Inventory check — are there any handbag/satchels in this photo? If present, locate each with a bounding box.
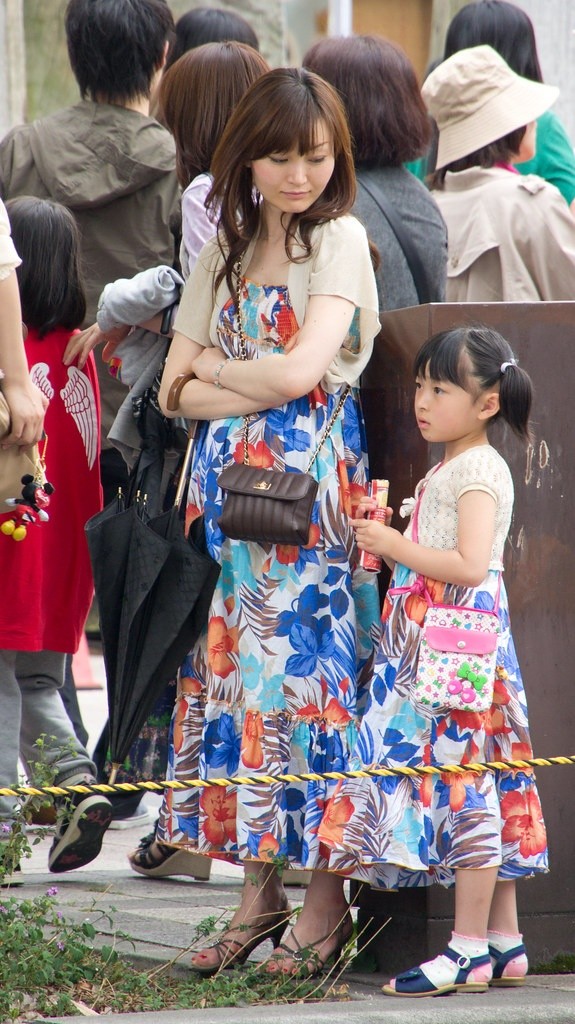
[215,463,319,546]
[414,604,503,712]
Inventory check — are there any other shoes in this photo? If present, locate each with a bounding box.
[0,855,24,885]
[108,801,149,830]
[48,777,113,873]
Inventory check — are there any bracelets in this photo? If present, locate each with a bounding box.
[215,358,233,389]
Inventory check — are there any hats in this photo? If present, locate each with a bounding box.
[419,45,560,174]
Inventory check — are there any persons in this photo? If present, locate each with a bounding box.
[0,0,449,885]
[419,44,575,302]
[401,0,575,208]
[157,67,382,977]
[317,326,550,997]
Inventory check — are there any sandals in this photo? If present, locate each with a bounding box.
[381,943,527,997]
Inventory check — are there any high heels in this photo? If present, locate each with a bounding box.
[129,837,213,881]
[190,895,293,974]
[258,906,354,980]
